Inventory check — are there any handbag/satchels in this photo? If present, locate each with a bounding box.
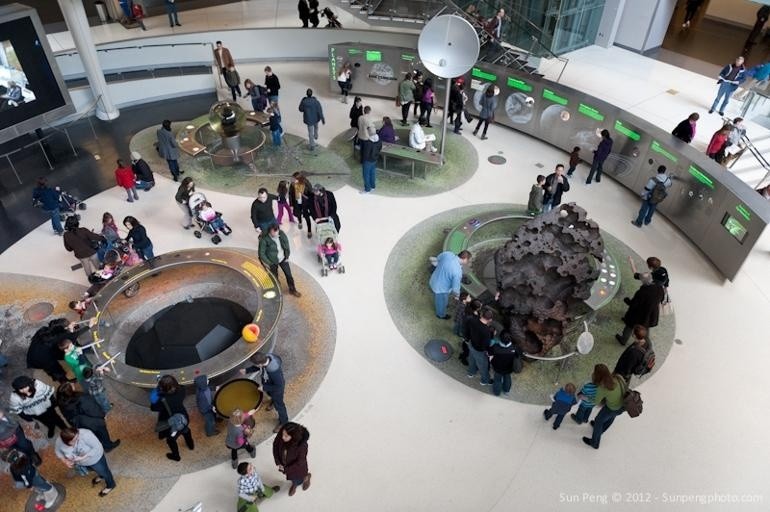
[32,198,44,208]
[659,300,673,317]
[625,388,643,417]
[155,422,169,432]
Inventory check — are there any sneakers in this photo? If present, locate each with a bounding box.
[49,427,55,438]
[459,353,509,396]
[544,408,595,429]
[99,488,112,497]
[437,313,450,318]
[92,476,103,485]
[272,486,280,491]
[207,430,219,436]
[616,334,624,344]
[265,403,273,410]
[273,424,281,433]
[289,219,313,239]
[105,439,120,454]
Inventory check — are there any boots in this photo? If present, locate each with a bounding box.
[36,485,58,509]
[473,128,478,136]
[251,447,255,458]
[232,459,238,469]
[481,133,488,139]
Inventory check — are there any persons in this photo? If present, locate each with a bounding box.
[722,118,744,167]
[744,5,770,53]
[707,125,731,159]
[683,0,703,29]
[709,56,746,116]
[672,113,700,144]
[733,62,770,100]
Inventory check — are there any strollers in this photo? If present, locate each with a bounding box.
[319,7,343,28]
[86,239,141,300]
[184,191,232,244]
[312,215,347,278]
[52,188,88,223]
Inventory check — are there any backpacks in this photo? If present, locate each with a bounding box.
[648,178,667,206]
[637,338,657,376]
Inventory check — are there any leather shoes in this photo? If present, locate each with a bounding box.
[583,437,591,445]
[302,474,311,490]
[166,453,181,461]
[287,484,297,496]
[289,289,300,296]
